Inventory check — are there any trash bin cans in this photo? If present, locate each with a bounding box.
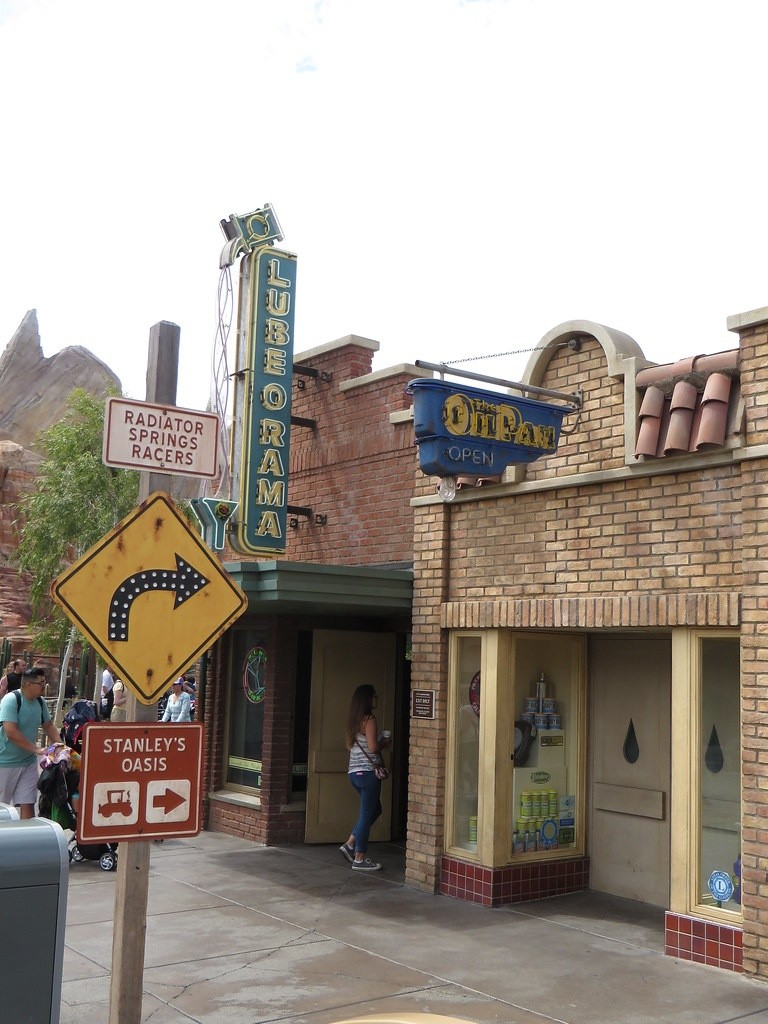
[0,800,68,1024]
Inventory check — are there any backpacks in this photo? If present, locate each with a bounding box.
[100,681,124,718]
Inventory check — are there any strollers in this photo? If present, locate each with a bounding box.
[36,760,118,872]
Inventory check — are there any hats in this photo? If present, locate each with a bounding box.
[174,677,183,684]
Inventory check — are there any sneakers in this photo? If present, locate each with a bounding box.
[339,843,355,864]
[352,858,382,872]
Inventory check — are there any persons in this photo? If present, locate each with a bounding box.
[71,777,80,814]
[339,684,391,870]
[456,687,479,794]
[0,658,199,722]
[0,668,65,819]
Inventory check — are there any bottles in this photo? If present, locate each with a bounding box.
[733,854,741,904]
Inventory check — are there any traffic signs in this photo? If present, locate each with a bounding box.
[76,722,206,845]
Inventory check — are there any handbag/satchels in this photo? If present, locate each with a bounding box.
[375,765,389,778]
[37,768,57,795]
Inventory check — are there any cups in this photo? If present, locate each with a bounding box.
[382,731,391,738]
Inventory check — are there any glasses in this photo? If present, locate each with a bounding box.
[374,695,378,698]
[29,680,46,686]
